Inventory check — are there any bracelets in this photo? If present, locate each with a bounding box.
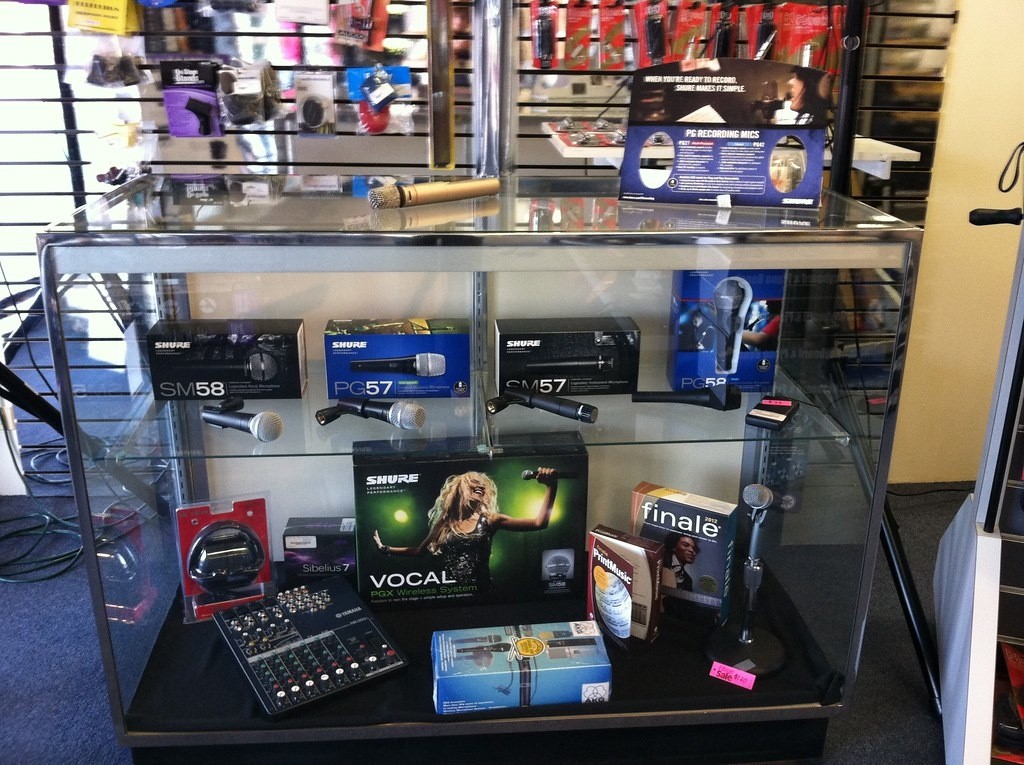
[381,545,390,555]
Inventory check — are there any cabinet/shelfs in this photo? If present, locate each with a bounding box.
[38,0,924,765]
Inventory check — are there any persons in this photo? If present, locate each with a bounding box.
[787,64,828,124]
[372,466,559,593]
[661,530,700,592]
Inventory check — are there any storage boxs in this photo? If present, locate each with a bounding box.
[153,319,310,401]
[431,618,610,715]
[323,316,470,401]
[665,268,788,393]
[587,524,667,643]
[283,518,356,577]
[618,57,829,213]
[495,315,642,399]
[630,479,740,628]
[352,428,589,617]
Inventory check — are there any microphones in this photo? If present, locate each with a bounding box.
[522,470,582,480]
[743,484,782,509]
[457,643,511,653]
[336,396,424,430]
[712,276,753,374]
[351,352,446,377]
[201,406,283,442]
[527,355,613,374]
[502,386,598,423]
[174,353,278,380]
[452,635,501,646]
[367,177,500,209]
[761,80,778,120]
[699,576,716,597]
[632,384,742,411]
